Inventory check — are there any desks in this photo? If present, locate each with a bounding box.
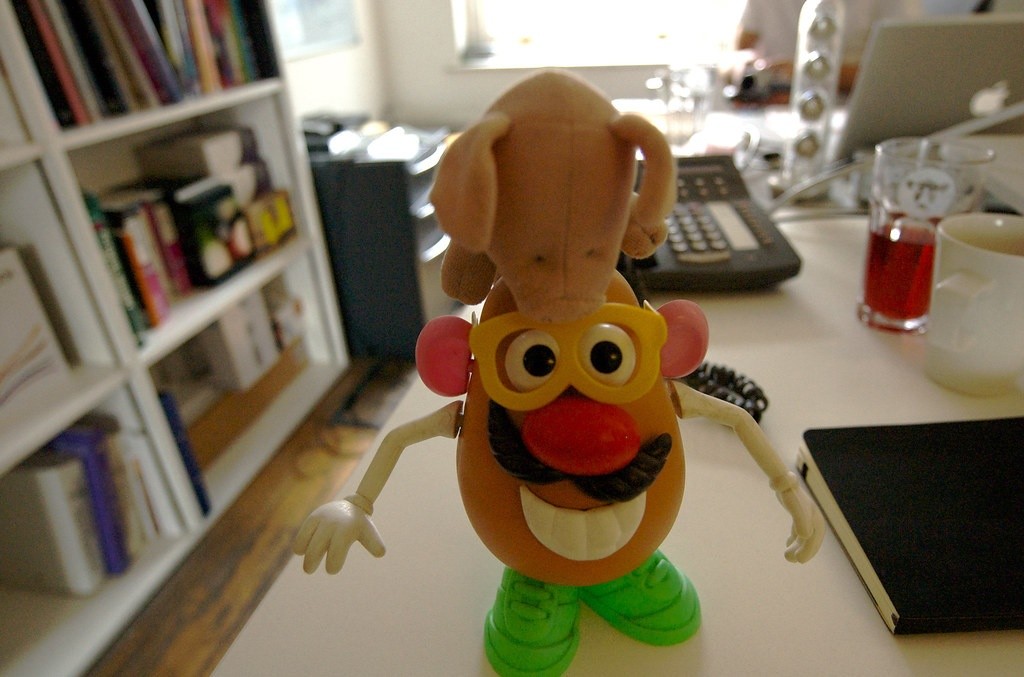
[206,98,1023,676]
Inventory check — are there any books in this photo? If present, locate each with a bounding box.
[1,0,297,601]
[796,416,1024,635]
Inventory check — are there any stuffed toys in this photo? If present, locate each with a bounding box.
[424,69,679,320]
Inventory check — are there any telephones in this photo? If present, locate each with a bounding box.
[613,155,800,306]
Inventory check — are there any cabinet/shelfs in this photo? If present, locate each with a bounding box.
[1,1,350,676]
[309,127,451,362]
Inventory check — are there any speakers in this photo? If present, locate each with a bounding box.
[777,0,847,195]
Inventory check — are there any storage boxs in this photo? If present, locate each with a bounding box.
[185,340,311,469]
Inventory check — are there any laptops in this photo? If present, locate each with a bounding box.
[770,13,1024,164]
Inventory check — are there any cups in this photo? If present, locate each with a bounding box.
[855,134,996,336]
[923,213,1024,397]
